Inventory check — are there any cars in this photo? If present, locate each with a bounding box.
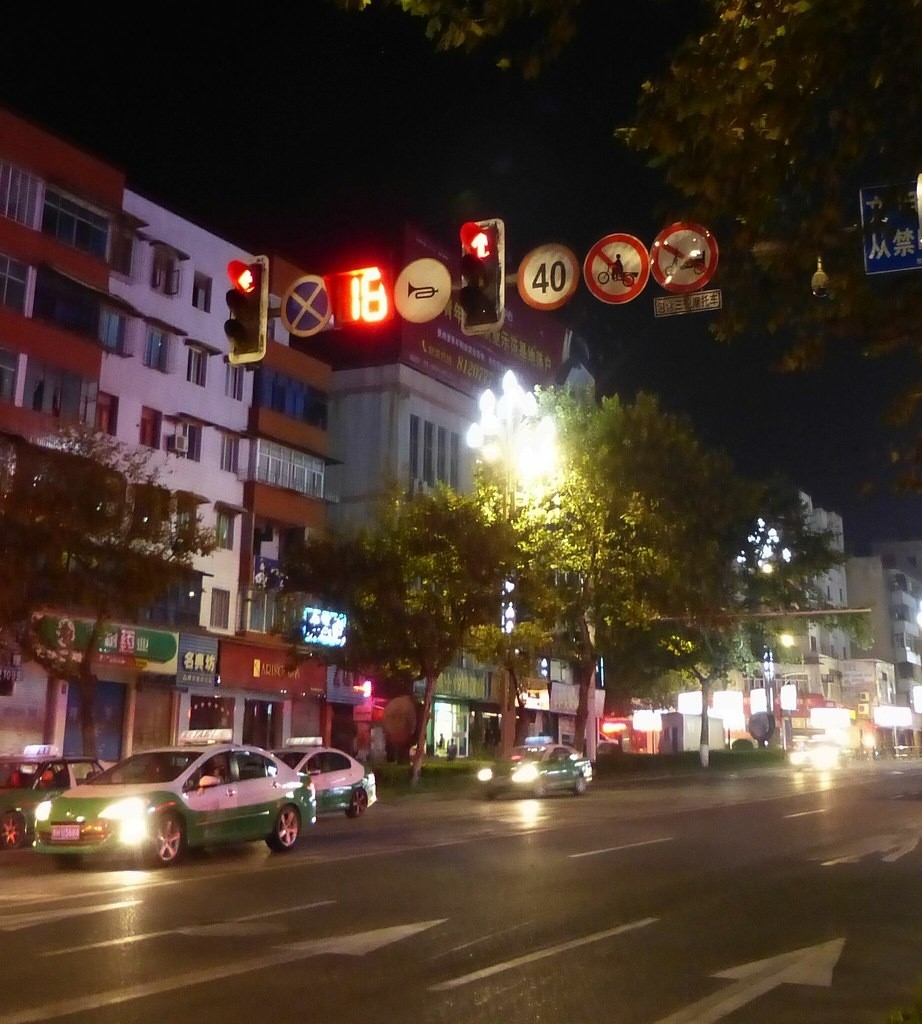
[793,741,847,770]
[478,735,592,798]
[264,736,377,818]
[32,728,318,868]
[0,745,108,850]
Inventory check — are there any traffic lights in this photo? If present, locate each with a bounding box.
[333,258,391,325]
[459,218,505,336]
[224,255,269,364]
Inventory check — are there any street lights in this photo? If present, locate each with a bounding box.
[737,519,794,747]
[467,369,561,749]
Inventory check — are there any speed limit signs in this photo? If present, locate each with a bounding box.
[518,243,580,311]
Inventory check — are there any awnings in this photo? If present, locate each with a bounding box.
[149,237,190,263]
[214,501,248,516]
[144,314,188,337]
[183,338,224,357]
[50,170,149,232]
[175,489,211,503]
[176,412,254,440]
[38,261,144,318]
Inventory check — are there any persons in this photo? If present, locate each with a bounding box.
[445,738,457,760]
[36,766,59,789]
